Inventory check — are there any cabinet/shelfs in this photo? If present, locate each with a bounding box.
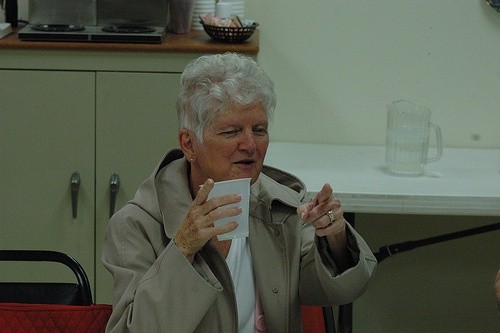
[0,53,258,309]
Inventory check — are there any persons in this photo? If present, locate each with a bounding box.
[102,51,378,333]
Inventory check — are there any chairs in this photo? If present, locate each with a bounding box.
[0,248,113,333]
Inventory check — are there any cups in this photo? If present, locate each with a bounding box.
[198,178,251,241]
[192,0,245,30]
[168,0,194,34]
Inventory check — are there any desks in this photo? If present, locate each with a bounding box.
[264,141,500,333]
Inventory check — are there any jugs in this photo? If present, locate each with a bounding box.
[384,97,443,177]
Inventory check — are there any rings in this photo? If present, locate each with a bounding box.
[326,209,337,224]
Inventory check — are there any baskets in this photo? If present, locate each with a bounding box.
[199,20,256,42]
